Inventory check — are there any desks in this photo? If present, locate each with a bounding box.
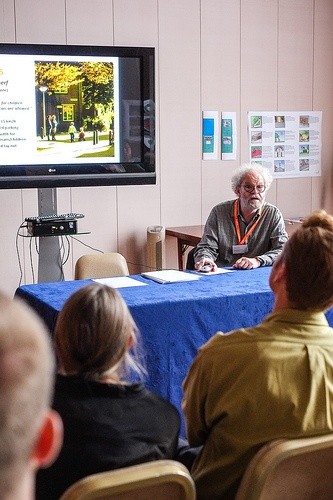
[166,223,302,271]
[14,265,333,441]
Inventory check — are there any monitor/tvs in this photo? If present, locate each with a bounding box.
[0,43,157,190]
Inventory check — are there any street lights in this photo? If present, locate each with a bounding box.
[39,86,48,138]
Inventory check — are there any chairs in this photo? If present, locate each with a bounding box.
[60,459,196,500]
[235,434,333,500]
[75,253,129,280]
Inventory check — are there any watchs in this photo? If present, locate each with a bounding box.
[257,257,263,267]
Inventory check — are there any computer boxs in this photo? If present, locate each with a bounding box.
[26,220,78,236]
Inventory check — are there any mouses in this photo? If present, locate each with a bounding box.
[201,265,212,272]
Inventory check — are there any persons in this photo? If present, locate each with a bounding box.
[46,114,87,143]
[177,210,333,500]
[194,162,288,270]
[0,284,181,500]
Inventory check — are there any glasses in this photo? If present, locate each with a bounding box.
[240,185,267,193]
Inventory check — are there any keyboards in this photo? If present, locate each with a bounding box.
[25,214,85,222]
